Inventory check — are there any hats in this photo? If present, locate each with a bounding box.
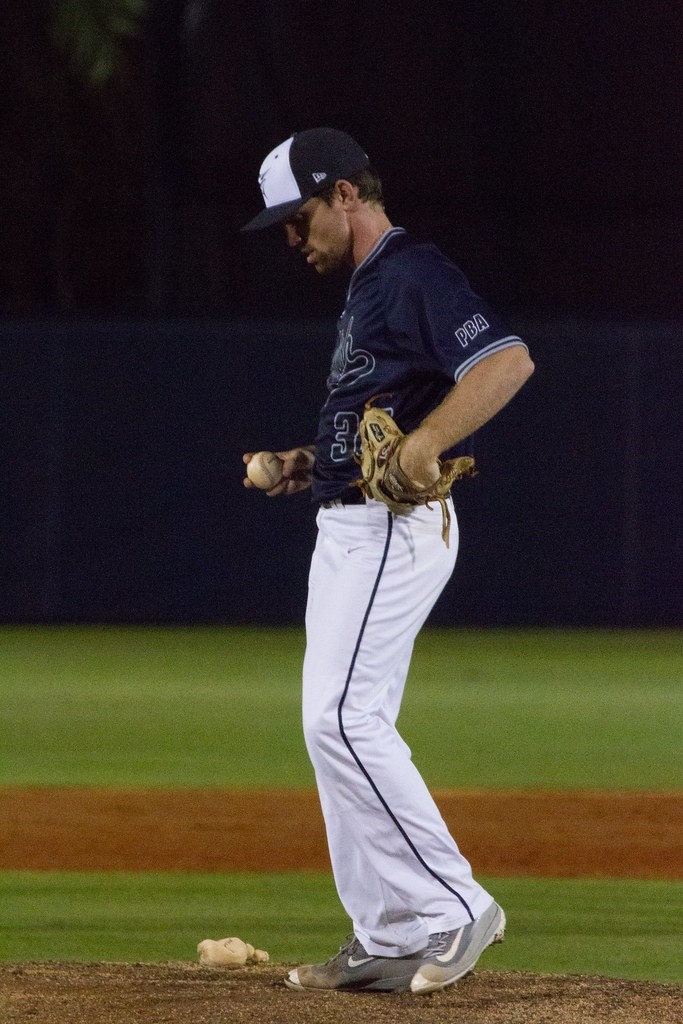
[240,128,370,232]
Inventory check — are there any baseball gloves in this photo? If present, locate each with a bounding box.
[356,406,477,518]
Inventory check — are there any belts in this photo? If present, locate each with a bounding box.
[322,494,418,508]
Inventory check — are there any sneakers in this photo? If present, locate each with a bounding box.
[283,933,427,994]
[411,901,507,994]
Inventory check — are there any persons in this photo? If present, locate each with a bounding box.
[243,128,538,998]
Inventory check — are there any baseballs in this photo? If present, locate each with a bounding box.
[246,450,285,491]
